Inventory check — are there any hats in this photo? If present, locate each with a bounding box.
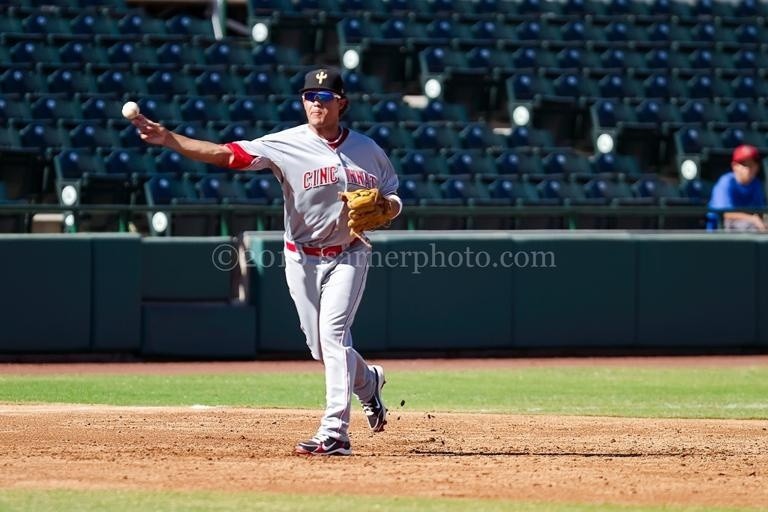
[733,145,759,162]
[299,69,344,97]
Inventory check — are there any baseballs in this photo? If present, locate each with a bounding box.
[120,101,139,120]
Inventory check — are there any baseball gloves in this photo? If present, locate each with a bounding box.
[337,188,393,234]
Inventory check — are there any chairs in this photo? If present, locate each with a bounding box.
[0,0,768,205]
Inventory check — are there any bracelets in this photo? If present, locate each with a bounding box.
[387,195,403,220]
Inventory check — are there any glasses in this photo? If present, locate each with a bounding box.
[304,91,340,101]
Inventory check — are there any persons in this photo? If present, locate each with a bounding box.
[705,144,768,231]
[131,67,403,457]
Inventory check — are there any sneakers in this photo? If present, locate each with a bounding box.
[295,431,352,455]
[360,364,386,431]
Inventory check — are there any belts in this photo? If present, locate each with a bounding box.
[286,236,359,257]
